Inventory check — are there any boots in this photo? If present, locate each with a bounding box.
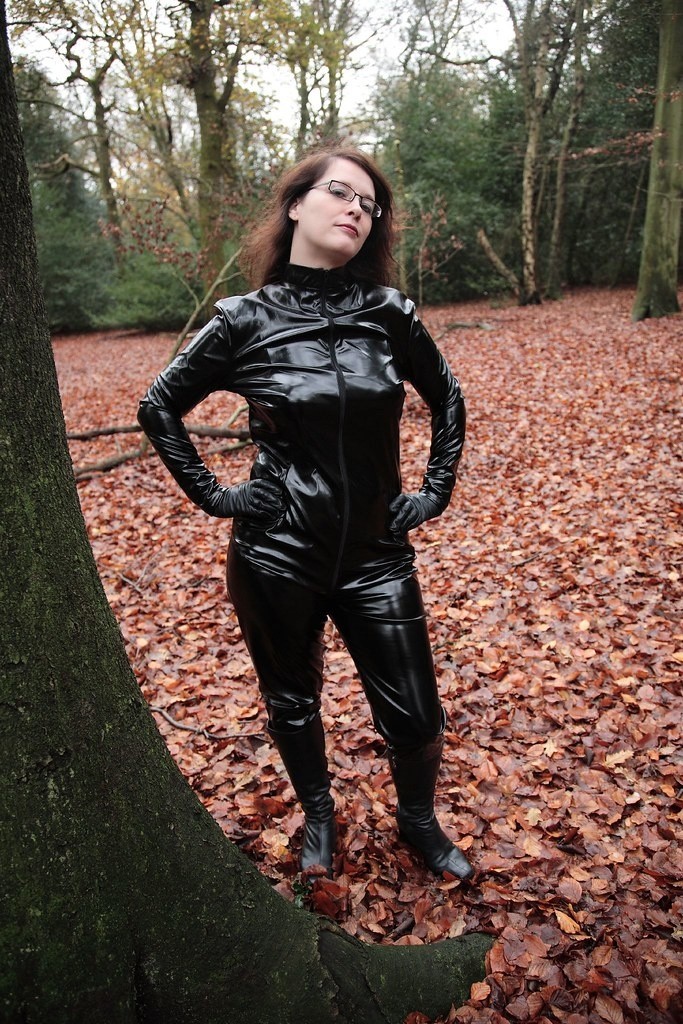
[265,712,335,889]
[385,742,474,880]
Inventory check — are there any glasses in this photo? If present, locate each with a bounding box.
[303,180,382,217]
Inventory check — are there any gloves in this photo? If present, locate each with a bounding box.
[388,489,445,541]
[211,479,288,522]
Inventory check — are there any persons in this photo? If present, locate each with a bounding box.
[136,149,476,883]
